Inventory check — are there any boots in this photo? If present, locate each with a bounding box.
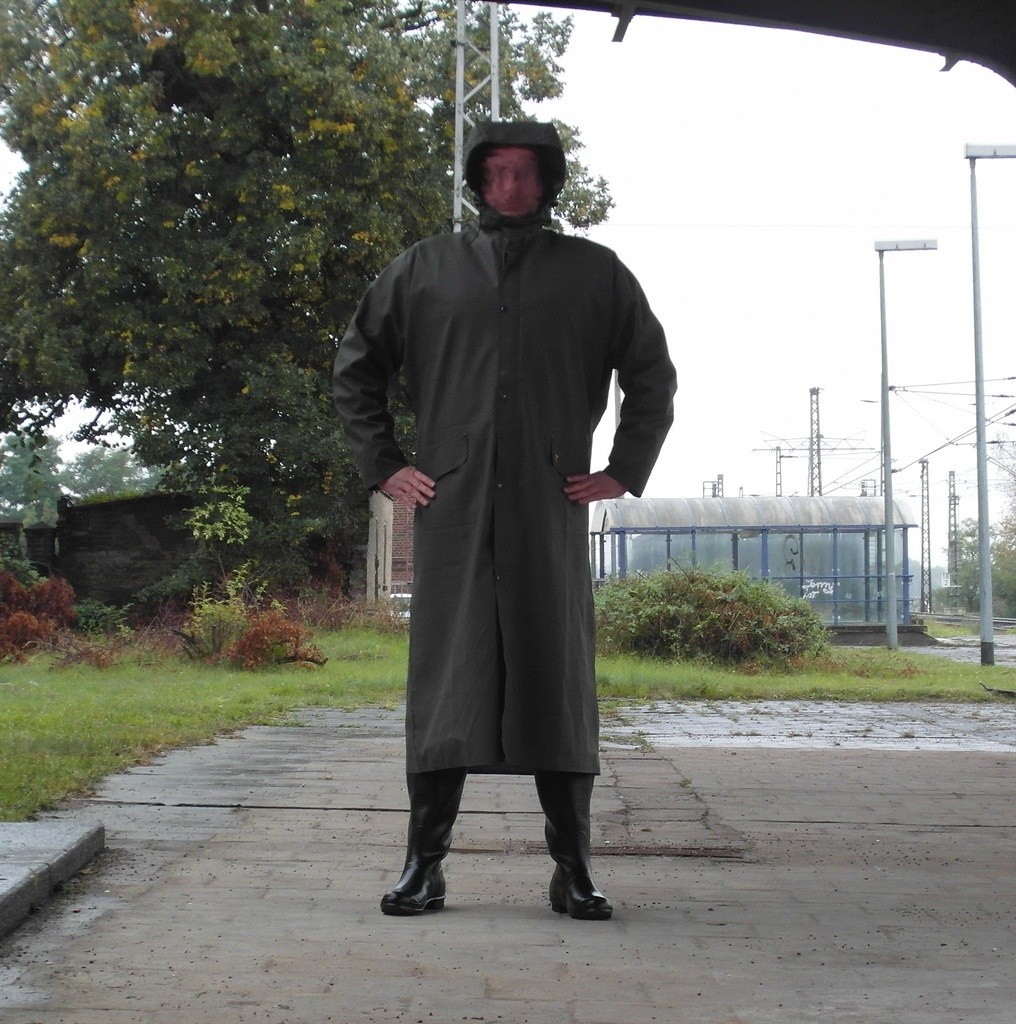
[535,772,613,919]
[381,768,469,916]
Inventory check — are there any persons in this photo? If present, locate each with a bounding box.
[333,121,678,920]
